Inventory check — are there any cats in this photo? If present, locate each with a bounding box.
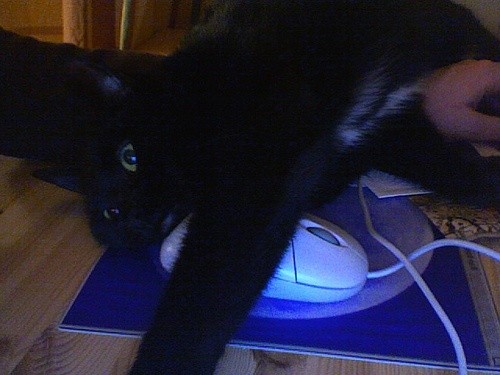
[31,0,499,375]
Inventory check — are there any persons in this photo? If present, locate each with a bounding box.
[1,25,500,170]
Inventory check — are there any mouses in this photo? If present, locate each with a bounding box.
[160,211,369,304]
